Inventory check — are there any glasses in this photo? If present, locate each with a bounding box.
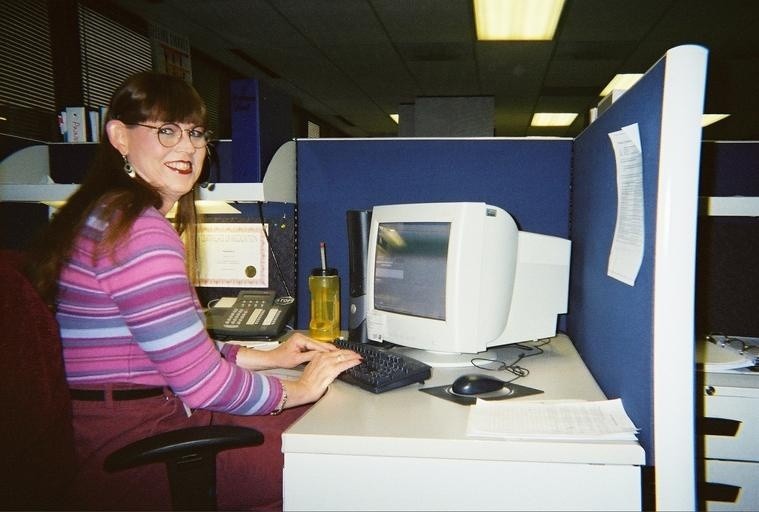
[124,121,209,149]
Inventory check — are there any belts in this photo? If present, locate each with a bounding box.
[69,385,173,402]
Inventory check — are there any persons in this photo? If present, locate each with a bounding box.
[24,68,362,512]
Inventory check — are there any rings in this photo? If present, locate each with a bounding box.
[336,354,343,361]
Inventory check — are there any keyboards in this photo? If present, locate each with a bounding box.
[301,339,431,394]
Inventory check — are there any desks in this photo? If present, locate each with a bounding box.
[253,326,645,511]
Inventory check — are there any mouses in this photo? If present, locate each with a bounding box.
[453,377,505,396]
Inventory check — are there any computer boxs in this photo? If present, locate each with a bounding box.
[346,209,374,344]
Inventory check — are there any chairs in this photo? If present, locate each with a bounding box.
[1,250,264,512]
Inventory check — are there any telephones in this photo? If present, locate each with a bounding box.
[205,290,297,339]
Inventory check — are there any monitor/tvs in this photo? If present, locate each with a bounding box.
[367,202,571,368]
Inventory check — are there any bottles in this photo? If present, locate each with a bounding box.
[308,268,340,341]
